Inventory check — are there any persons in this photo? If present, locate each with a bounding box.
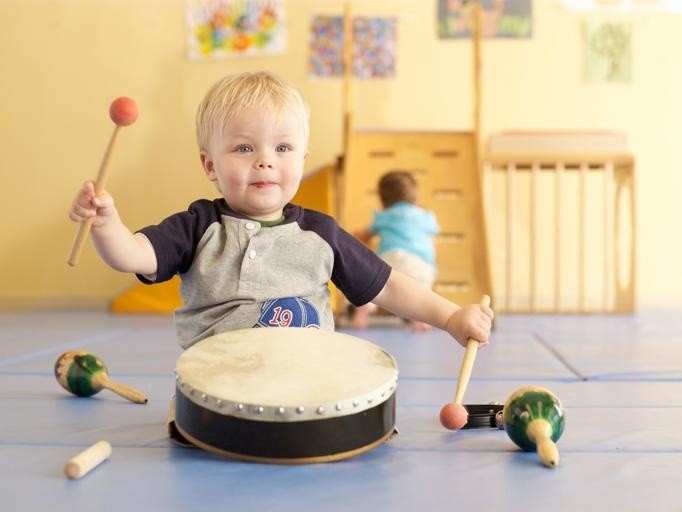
[69,71,494,448]
[345,171,442,332]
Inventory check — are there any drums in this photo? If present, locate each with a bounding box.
[176,327,397,462]
